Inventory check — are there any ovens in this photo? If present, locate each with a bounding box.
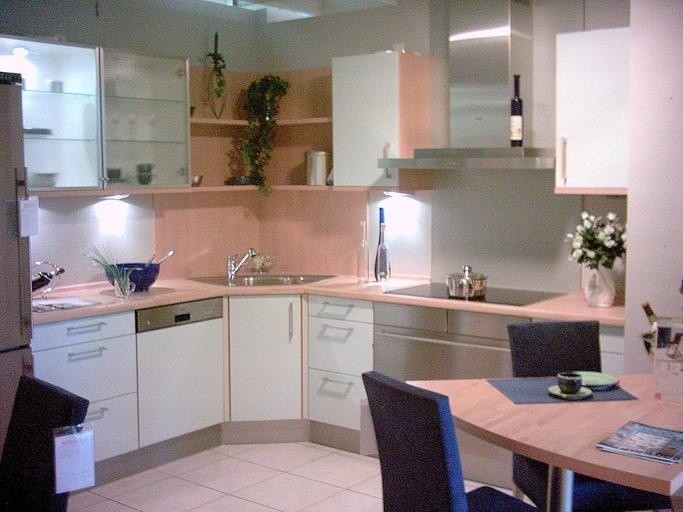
[374,303,532,489]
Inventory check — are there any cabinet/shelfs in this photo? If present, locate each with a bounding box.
[31,310,138,464]
[305,297,376,431]
[227,294,303,422]
[191,69,332,195]
[554,28,628,195]
[100,46,191,190]
[332,52,451,191]
[0,33,102,192]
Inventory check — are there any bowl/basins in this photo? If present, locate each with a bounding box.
[192,175,203,187]
[28,170,60,186]
[104,262,162,293]
[245,254,279,276]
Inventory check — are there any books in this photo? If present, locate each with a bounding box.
[593,418,683,467]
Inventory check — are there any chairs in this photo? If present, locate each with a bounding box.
[0,374,90,511]
[361,370,541,512]
[506,320,612,512]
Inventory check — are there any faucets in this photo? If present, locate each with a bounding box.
[223,246,257,286]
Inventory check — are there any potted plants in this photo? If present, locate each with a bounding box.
[228,74,291,199]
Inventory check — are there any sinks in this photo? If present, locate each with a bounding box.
[236,273,293,286]
[294,272,336,286]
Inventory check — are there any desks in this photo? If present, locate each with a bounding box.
[404,376,683,512]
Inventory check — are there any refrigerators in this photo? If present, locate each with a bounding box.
[1,71,36,456]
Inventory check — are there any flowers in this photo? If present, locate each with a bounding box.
[563,210,628,269]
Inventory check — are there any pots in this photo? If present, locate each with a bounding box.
[444,263,489,302]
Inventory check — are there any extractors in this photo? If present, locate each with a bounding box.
[376,1,557,173]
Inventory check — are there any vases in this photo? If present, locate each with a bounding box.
[584,265,616,308]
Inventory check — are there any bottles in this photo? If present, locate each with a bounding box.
[641,301,663,333]
[509,73,524,147]
[31,264,66,293]
[355,219,371,286]
[375,207,392,285]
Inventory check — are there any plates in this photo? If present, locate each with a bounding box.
[573,370,620,392]
[547,384,593,401]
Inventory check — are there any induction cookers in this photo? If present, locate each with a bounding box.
[384,281,570,307]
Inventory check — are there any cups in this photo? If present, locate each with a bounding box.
[305,150,330,186]
[106,159,159,185]
[557,370,582,395]
[50,80,63,93]
